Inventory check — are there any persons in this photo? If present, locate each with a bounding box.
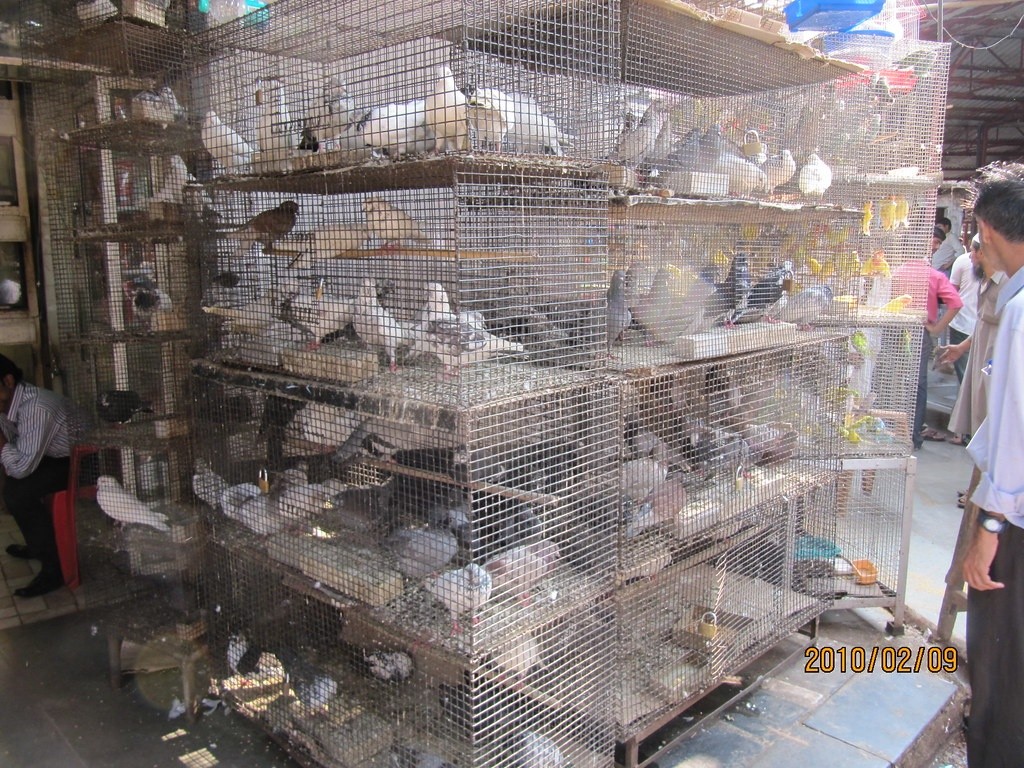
[963,162,1024,766]
[0,354,73,599]
[838,215,1011,515]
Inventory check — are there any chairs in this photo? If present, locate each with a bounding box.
[53,444,99,589]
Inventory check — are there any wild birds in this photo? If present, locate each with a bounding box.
[668,190,918,447]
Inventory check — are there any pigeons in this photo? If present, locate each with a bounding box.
[91,58,842,729]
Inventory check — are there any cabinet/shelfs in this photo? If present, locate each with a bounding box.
[0,55,110,417]
[182,0,618,768]
[17,0,235,663]
[613,0,847,741]
[694,28,953,635]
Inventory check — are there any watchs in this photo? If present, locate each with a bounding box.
[976,508,1006,535]
